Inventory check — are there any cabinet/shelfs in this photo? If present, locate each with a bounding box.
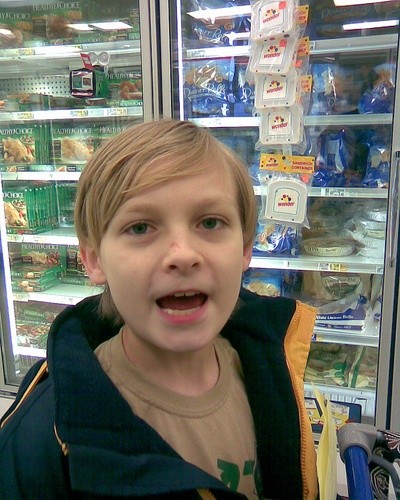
[0,0,400,444]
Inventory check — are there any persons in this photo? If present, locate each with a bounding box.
[0,119,319,500]
